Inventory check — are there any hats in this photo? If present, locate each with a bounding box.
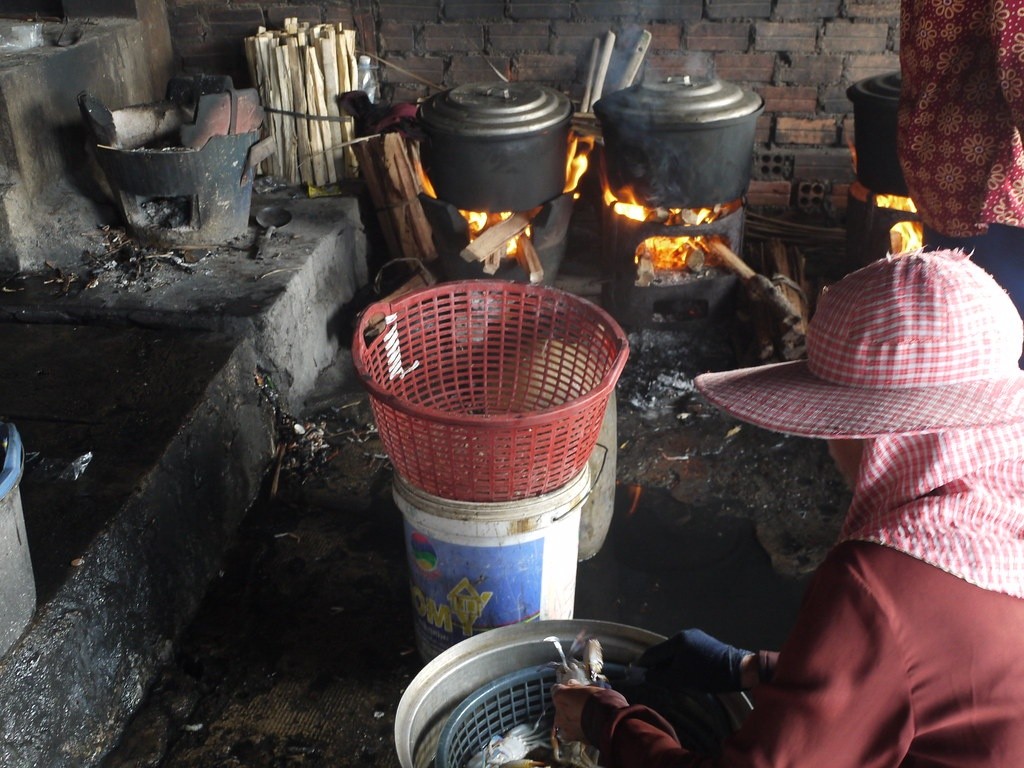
[693,246,1024,440]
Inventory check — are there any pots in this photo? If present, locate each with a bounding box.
[418,77,572,213]
[589,71,765,212]
[843,71,931,198]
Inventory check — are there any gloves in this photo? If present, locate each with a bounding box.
[636,627,753,699]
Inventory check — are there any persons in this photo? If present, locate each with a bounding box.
[552,245,1024,768]
[900,0,1024,370]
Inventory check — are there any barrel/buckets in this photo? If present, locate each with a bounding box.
[389,438,614,677]
[392,620,773,768]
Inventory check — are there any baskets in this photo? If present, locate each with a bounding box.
[352,277,632,502]
[434,661,630,768]
